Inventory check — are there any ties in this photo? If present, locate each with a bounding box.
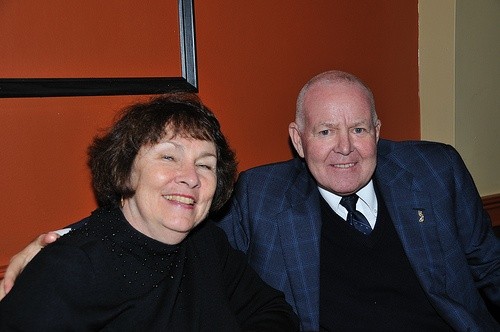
[338,193,374,236]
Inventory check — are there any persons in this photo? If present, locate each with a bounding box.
[0,71,500,331]
[0,89,305,332]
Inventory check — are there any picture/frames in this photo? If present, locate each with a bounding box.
[0,0,201,98]
